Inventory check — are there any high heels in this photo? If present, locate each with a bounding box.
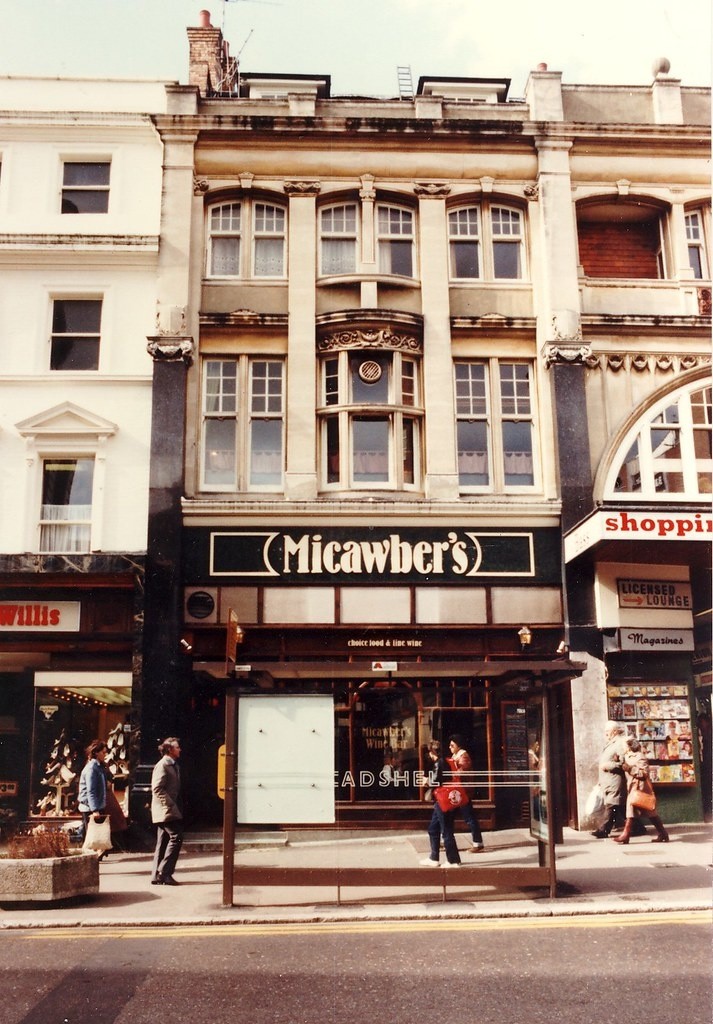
[652,833,669,842]
[614,834,629,844]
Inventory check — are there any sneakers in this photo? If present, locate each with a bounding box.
[441,861,459,868]
[419,858,438,866]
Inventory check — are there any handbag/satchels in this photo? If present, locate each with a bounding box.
[82,814,112,850]
[433,780,469,812]
[587,784,605,813]
[631,778,656,811]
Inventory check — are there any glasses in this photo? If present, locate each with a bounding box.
[449,744,459,747]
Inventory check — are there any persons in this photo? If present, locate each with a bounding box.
[426,741,461,872]
[528,740,544,821]
[81,740,114,862]
[631,720,692,759]
[384,740,402,774]
[149,736,185,889]
[615,740,670,847]
[591,722,646,840]
[440,736,485,853]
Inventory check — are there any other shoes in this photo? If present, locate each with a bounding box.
[151,871,180,885]
[612,827,623,832]
[631,825,647,835]
[439,843,446,851]
[591,829,607,838]
[469,846,483,852]
